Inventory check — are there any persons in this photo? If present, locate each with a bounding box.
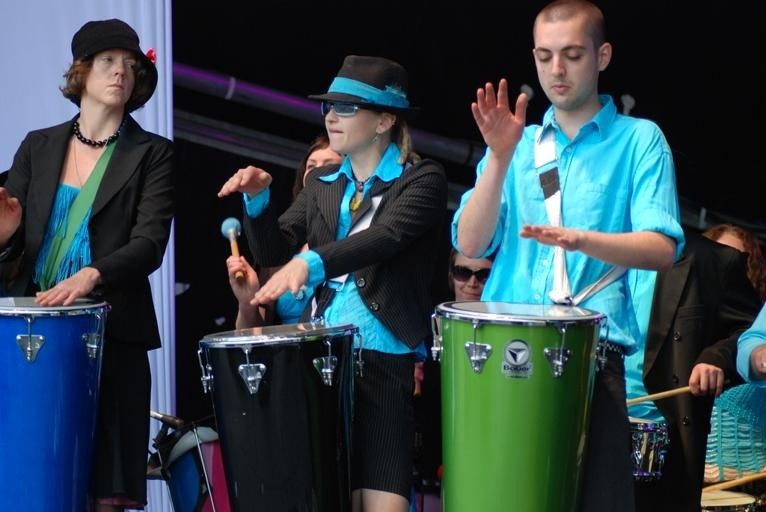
[641,221,766,512]
[414,247,497,486]
[445,2,687,510]
[0,18,188,508]
[215,52,446,512]
[291,132,342,200]
[226,241,314,331]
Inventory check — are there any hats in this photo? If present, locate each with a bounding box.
[309,55,408,110]
[71,18,158,114]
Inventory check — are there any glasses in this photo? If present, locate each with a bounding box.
[319,101,385,117]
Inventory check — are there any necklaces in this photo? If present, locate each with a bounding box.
[72,117,128,147]
[352,172,370,211]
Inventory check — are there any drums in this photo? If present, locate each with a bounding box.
[702,487,758,512]
[201,323,352,512]
[0,296,107,512]
[628,415,668,482]
[436,302,605,511]
